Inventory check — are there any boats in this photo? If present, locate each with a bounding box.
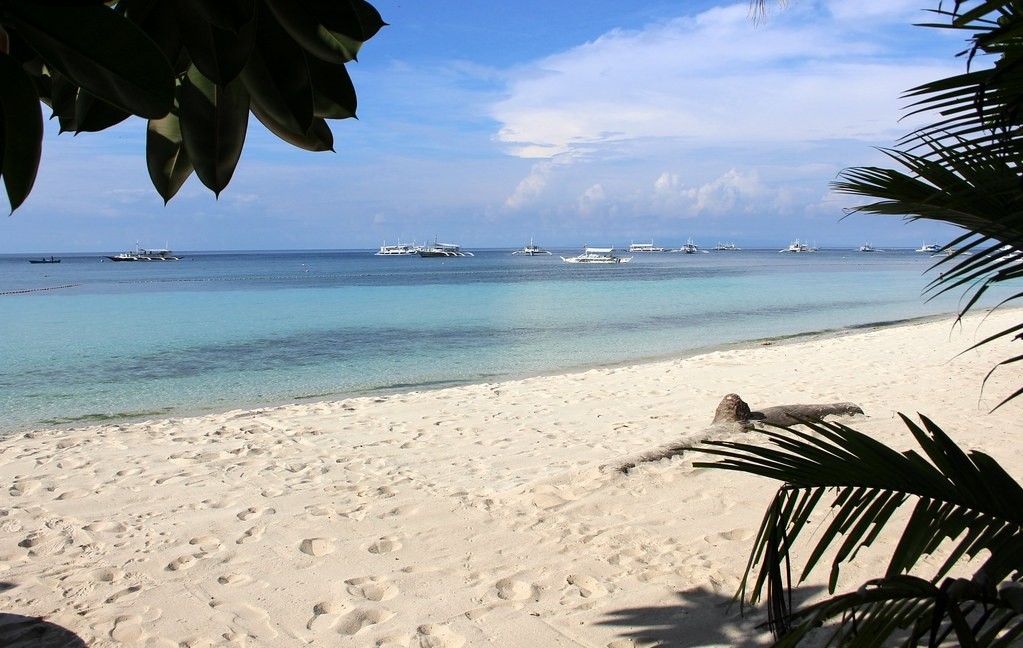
[916,241,942,252]
[374,234,475,257]
[778,236,819,253]
[711,240,742,251]
[103,240,184,261]
[511,237,553,256]
[671,235,699,254]
[29,260,61,263]
[560,243,633,264]
[627,239,665,252]
[854,245,876,252]
